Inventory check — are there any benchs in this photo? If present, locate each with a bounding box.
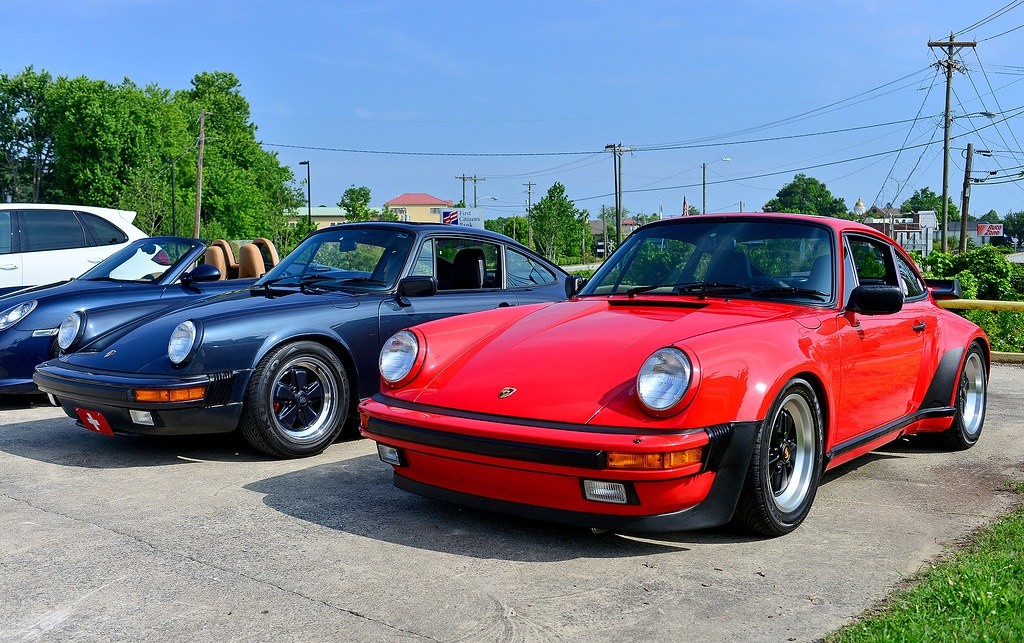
[776,278,887,291]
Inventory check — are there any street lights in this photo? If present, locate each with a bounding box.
[298,159,312,234]
[473,197,498,208]
[604,143,622,251]
[702,157,730,216]
[938,112,996,252]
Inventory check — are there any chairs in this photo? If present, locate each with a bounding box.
[701,252,754,291]
[452,249,487,290]
[238,244,265,278]
[205,246,227,280]
[385,251,408,285]
[804,255,832,301]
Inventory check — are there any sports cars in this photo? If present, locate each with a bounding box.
[32,222,589,462]
[364,210,993,540]
[0,237,352,407]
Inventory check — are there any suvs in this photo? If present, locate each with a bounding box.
[0,201,183,291]
